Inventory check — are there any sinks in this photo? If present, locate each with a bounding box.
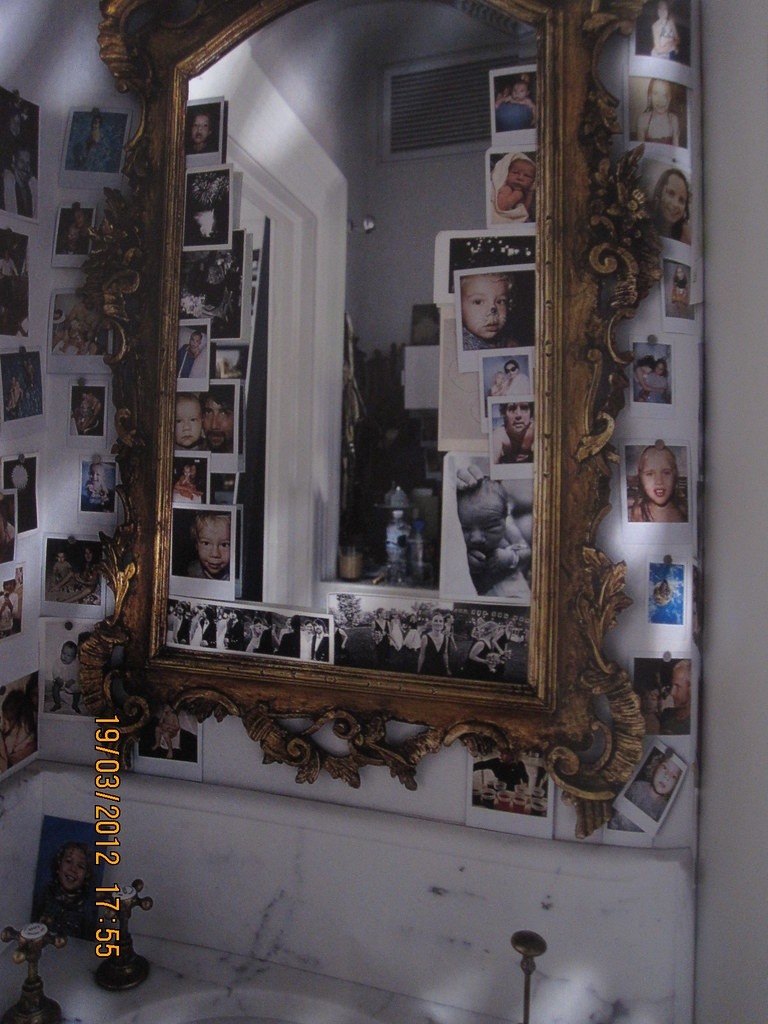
[113,989,381,1024]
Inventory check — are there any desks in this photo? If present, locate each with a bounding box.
[337,553,435,590]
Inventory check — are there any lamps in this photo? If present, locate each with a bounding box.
[347,213,374,236]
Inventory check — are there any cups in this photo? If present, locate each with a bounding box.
[338,547,361,582]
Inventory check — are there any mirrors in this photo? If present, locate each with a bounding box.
[76,0,664,838]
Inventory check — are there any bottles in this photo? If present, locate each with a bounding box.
[382,476,430,584]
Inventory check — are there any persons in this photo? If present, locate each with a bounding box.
[76,389,101,437]
[5,356,36,411]
[638,0,690,320]
[0,250,20,321]
[52,298,106,357]
[184,113,215,156]
[31,831,104,940]
[0,673,41,774]
[61,209,91,254]
[50,641,83,713]
[624,553,691,822]
[0,107,38,221]
[173,328,236,587]
[75,116,112,172]
[168,599,528,685]
[84,464,110,512]
[456,464,531,592]
[0,495,23,638]
[51,546,101,606]
[152,704,180,758]
[634,356,670,403]
[626,445,687,523]
[461,75,538,350]
[474,752,529,792]
[491,360,534,464]
